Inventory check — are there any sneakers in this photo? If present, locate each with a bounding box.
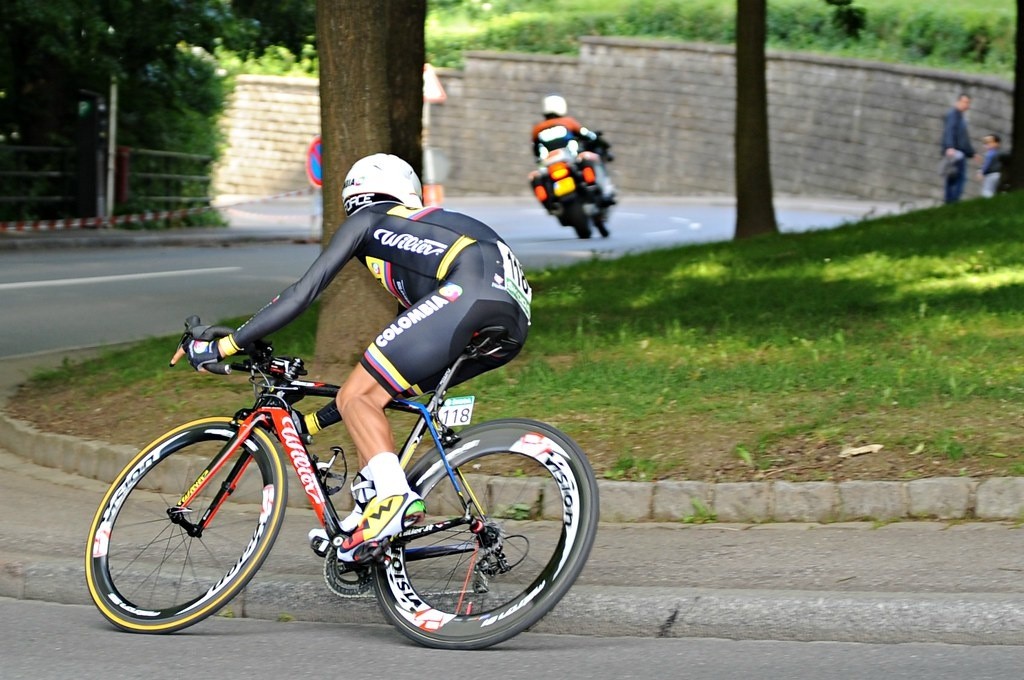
[307,503,368,545]
[335,491,426,566]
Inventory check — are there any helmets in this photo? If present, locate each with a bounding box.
[341,153,423,216]
[541,96,568,118]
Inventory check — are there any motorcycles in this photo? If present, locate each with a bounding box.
[527,125,619,241]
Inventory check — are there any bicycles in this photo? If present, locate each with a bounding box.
[84,315,600,651]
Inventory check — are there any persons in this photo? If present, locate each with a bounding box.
[974,134,1005,197]
[532,95,616,203]
[941,92,975,204]
[169,154,533,563]
